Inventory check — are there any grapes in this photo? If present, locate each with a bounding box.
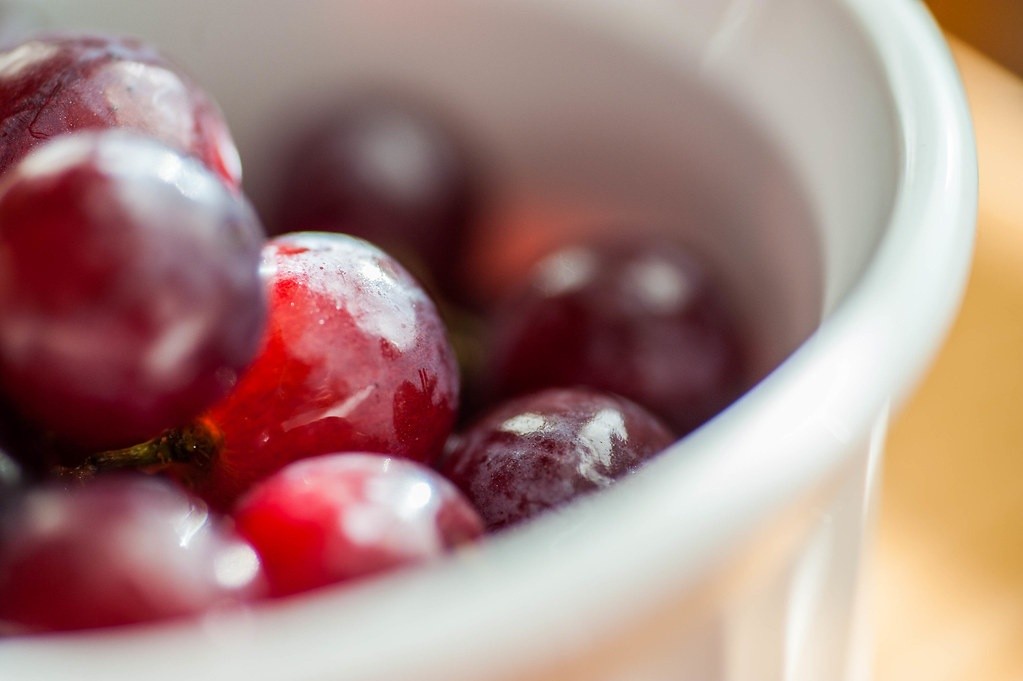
[0,40,736,640]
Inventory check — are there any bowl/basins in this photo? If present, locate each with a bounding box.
[1,1,979,681]
